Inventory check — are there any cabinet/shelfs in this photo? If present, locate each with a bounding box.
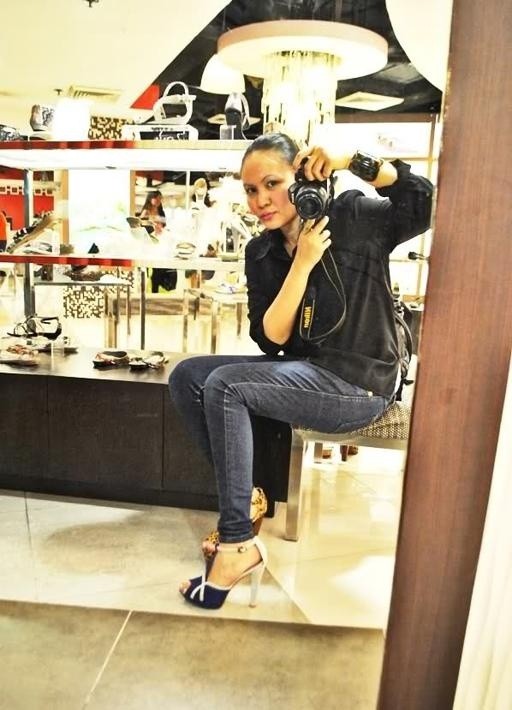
[311,112,440,296]
[0,140,292,518]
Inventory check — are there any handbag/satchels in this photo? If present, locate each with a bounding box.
[135,213,154,235]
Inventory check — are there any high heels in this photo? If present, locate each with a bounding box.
[201,488,268,563]
[179,536,267,610]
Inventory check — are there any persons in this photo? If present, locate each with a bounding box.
[170,132,434,606]
[139,191,166,233]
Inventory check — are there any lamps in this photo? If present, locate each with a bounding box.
[217,19,389,146]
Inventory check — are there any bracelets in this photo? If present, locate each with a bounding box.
[348,152,381,181]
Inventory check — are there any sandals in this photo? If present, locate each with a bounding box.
[6,211,59,254]
[7,313,62,341]
[214,271,248,295]
[32,336,79,354]
[0,344,43,367]
[190,178,208,218]
[223,90,250,139]
[92,350,169,372]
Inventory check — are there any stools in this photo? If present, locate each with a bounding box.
[282,400,415,543]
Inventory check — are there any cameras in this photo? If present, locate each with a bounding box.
[288,157,328,228]
